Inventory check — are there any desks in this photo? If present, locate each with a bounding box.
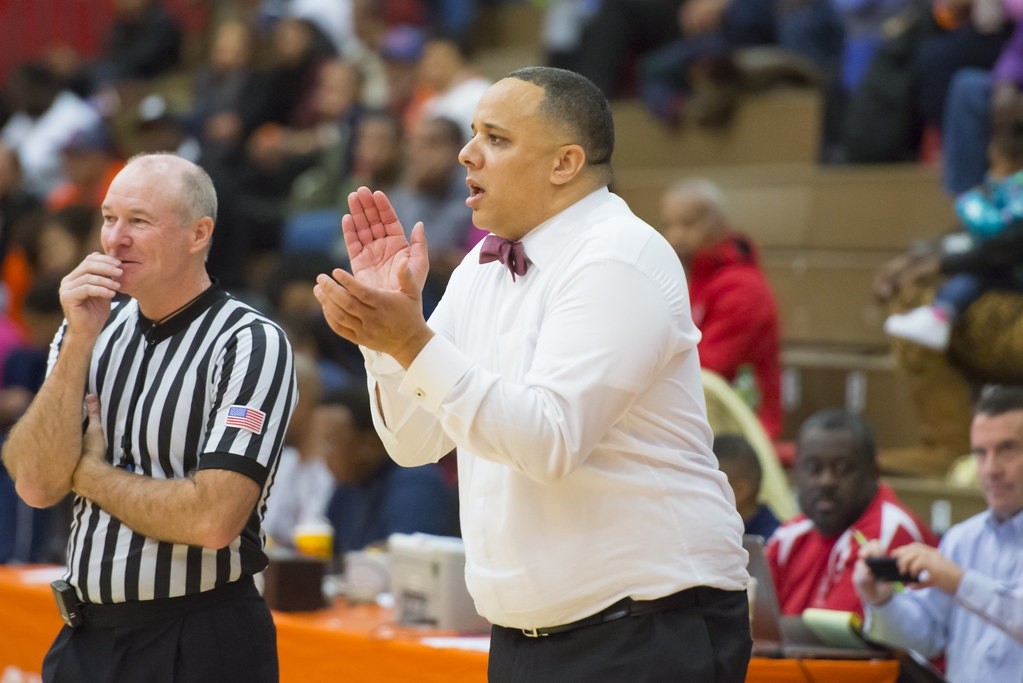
[0,564,898,683]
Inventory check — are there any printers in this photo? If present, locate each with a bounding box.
[387,532,492,635]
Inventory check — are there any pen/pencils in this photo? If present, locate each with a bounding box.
[855,531,904,593]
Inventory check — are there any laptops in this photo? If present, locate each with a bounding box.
[742,534,891,659]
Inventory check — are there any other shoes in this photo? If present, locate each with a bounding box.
[884,302,961,353]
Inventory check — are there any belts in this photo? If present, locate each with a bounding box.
[520,592,733,638]
[78,578,255,633]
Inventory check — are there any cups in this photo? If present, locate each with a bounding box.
[292,527,336,570]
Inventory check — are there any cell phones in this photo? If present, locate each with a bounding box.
[866,555,919,584]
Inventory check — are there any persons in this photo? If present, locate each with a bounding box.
[850,385,1023,683]
[530,0,1023,357]
[711,433,779,542]
[759,405,946,674]
[664,178,783,440]
[312,66,753,683]
[0,152,300,683]
[0,0,495,571]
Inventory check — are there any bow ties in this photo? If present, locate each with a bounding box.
[479,235,529,282]
